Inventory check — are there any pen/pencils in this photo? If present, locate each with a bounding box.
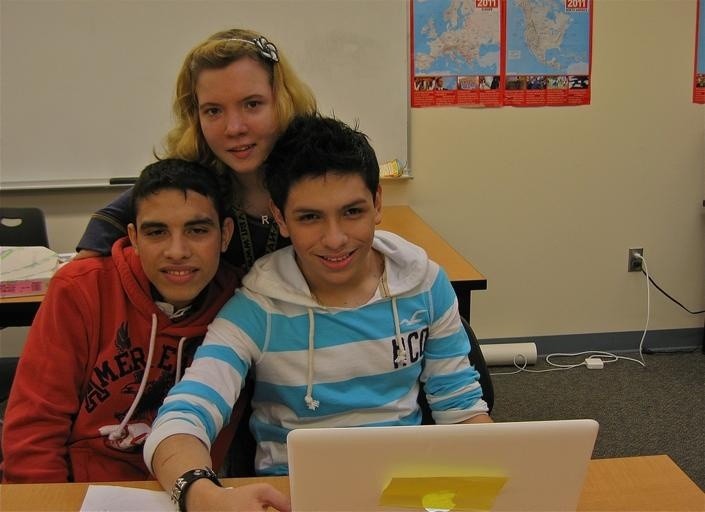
[109,177,138,185]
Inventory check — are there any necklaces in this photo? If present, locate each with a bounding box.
[244,200,271,225]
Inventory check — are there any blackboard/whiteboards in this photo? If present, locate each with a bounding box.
[0,0,412,190]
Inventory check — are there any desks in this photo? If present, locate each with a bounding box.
[0,453,705,512]
[0,205,488,326]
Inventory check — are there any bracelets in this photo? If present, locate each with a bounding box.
[169,465,222,511]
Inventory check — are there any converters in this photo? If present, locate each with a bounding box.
[584,357,604,371]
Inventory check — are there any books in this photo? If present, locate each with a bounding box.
[0,246,60,298]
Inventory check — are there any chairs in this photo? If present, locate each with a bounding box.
[417,314,493,425]
[0,207,49,329]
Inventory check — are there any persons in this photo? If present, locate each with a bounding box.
[414,77,437,91]
[0,152,251,486]
[69,29,320,273]
[140,112,495,511]
[435,77,449,91]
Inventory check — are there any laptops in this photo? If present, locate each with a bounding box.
[285,418,602,512]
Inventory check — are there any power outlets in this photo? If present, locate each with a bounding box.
[628,248,643,273]
